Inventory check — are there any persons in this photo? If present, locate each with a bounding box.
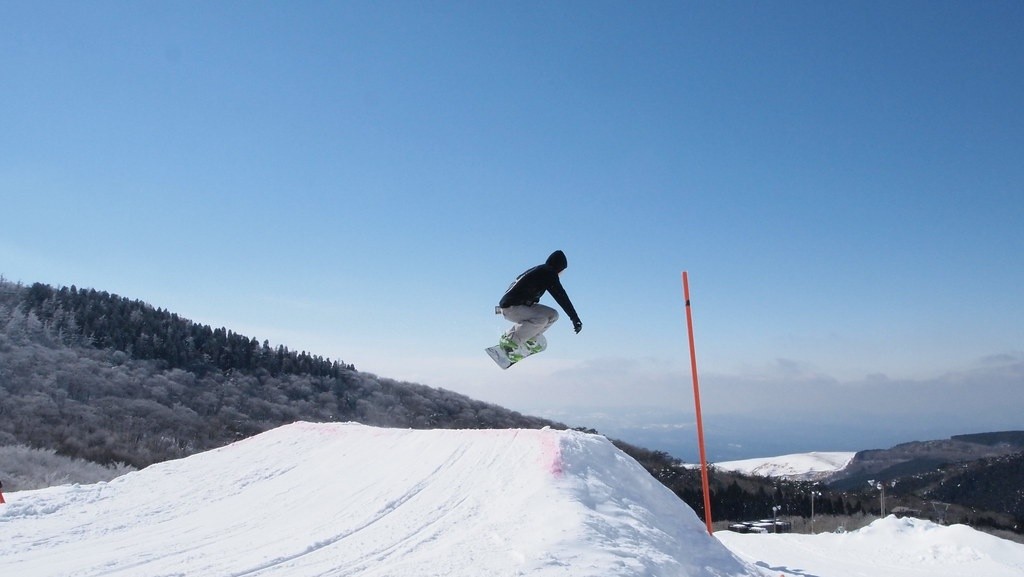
[499,251,583,363]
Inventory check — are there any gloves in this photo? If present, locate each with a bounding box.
[574,323,582,334]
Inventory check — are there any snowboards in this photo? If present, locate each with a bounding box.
[485,333,548,371]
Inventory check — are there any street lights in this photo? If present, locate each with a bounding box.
[773,507,776,533]
[877,484,885,519]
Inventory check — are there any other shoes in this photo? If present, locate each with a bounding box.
[500,337,523,361]
[525,340,543,352]
